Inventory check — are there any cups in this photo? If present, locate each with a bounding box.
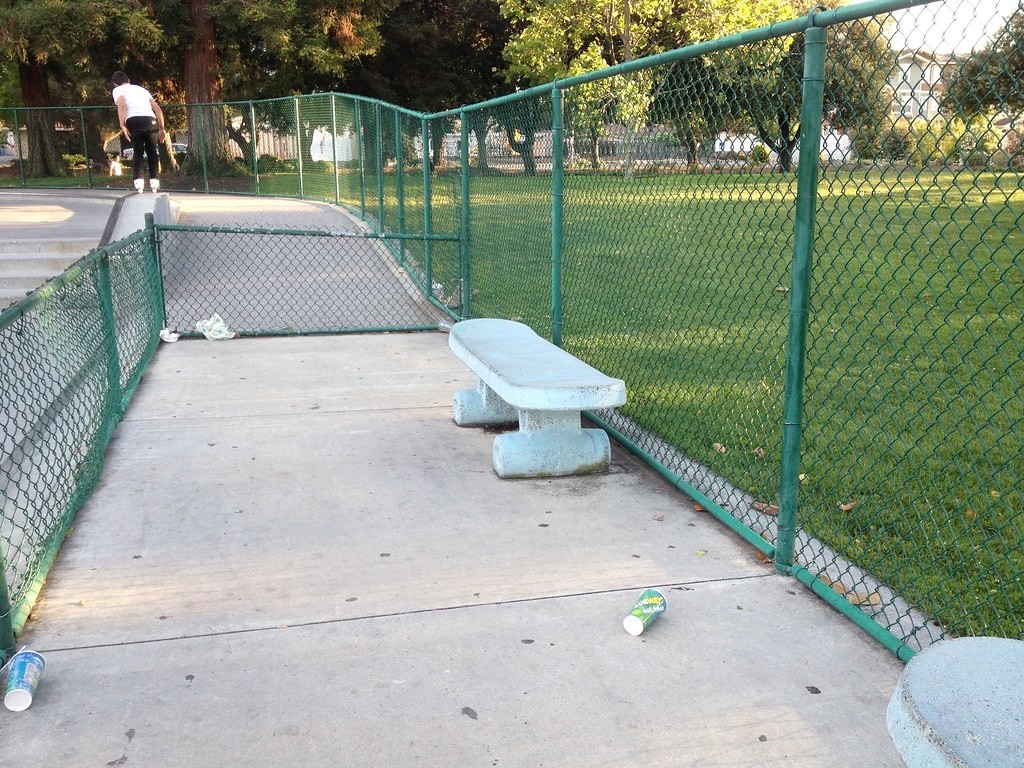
[4,651,46,712]
[622,588,668,636]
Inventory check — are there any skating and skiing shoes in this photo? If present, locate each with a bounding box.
[149,179,159,193]
[134,179,144,194]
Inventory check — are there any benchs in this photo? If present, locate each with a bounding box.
[448,319,626,475]
[884,636,1024,768]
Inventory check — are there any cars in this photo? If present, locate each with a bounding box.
[123,144,187,161]
[0,144,15,157]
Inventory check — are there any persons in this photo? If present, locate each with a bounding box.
[109,154,123,176]
[112,70,167,194]
